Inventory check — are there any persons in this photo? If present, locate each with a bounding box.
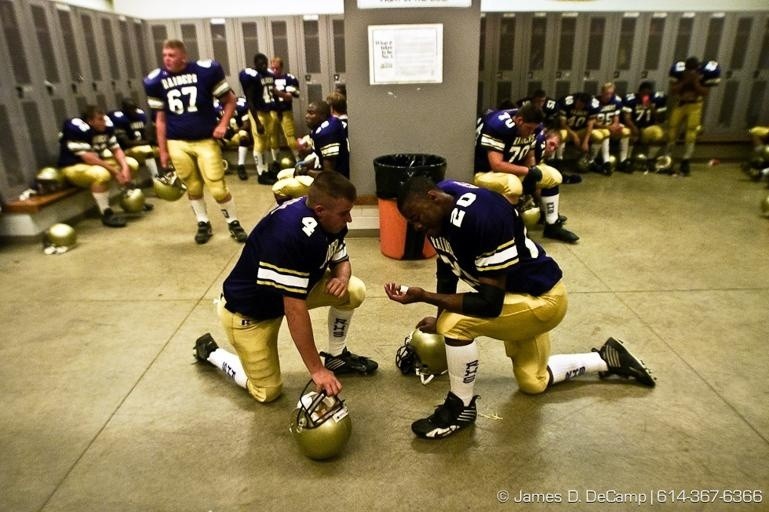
[474,56,722,242]
[57,39,349,243]
[193,168,378,404]
[385,175,656,439]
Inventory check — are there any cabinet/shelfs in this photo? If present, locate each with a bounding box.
[0,0,344,199]
[477,11,769,160]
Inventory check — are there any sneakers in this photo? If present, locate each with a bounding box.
[591,336,656,388]
[538,153,689,242]
[101,208,126,228]
[411,392,480,439]
[195,155,297,245]
[196,332,218,364]
[318,346,378,378]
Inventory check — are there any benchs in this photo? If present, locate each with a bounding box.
[0,186,97,239]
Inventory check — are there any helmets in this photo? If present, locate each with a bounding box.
[396,328,448,377]
[35,167,60,195]
[43,223,76,247]
[120,187,145,211]
[520,200,540,228]
[289,381,351,460]
[150,171,186,202]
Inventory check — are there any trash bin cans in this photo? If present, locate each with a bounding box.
[373,153,446,260]
